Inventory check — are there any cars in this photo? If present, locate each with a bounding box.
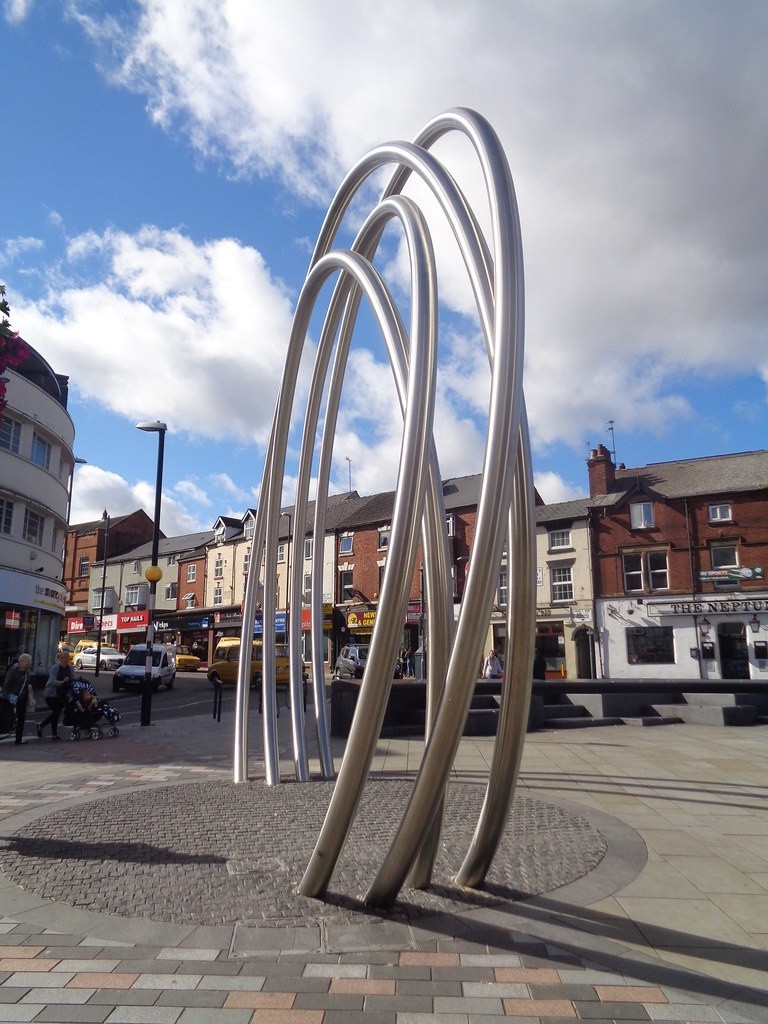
[173,645,200,672]
[58,639,129,671]
[207,636,305,687]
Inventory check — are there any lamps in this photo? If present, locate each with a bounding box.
[700,617,710,636]
[748,615,761,632]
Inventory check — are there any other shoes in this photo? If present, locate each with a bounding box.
[51,736,60,741]
[37,724,42,737]
[15,739,28,744]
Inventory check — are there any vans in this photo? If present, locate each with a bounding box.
[113,644,177,694]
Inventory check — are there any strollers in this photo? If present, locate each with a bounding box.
[62,674,122,742]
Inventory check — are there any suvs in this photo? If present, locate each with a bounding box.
[333,643,402,679]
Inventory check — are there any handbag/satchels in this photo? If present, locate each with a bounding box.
[0,692,18,705]
[63,701,85,726]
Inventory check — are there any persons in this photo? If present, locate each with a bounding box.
[533,649,547,680]
[482,650,503,680]
[0,653,32,746]
[120,645,128,654]
[79,691,118,718]
[35,651,72,742]
[394,643,417,681]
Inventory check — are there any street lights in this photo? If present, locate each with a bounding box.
[135,421,168,726]
[95,508,110,677]
[60,457,88,585]
[281,512,291,654]
